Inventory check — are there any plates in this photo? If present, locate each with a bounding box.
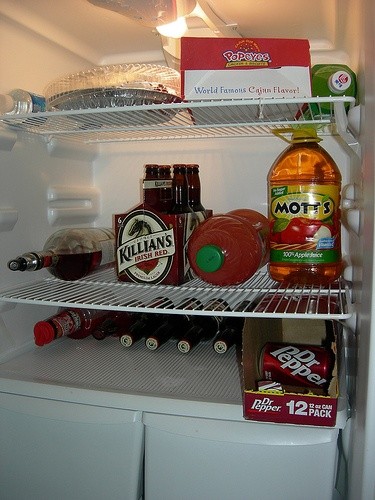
[47,90,181,124]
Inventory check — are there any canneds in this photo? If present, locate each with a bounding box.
[257,341,334,388]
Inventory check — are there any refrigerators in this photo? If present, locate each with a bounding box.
[0,0,375,500]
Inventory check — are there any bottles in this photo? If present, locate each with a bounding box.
[8,227,115,281]
[0,89,47,130]
[33,298,251,354]
[187,208,268,286]
[266,127,343,283]
[306,64,357,121]
[124,164,206,214]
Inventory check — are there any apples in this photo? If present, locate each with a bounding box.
[268,217,333,244]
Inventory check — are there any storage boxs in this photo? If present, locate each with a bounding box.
[241,317,340,426]
[180,35,313,125]
